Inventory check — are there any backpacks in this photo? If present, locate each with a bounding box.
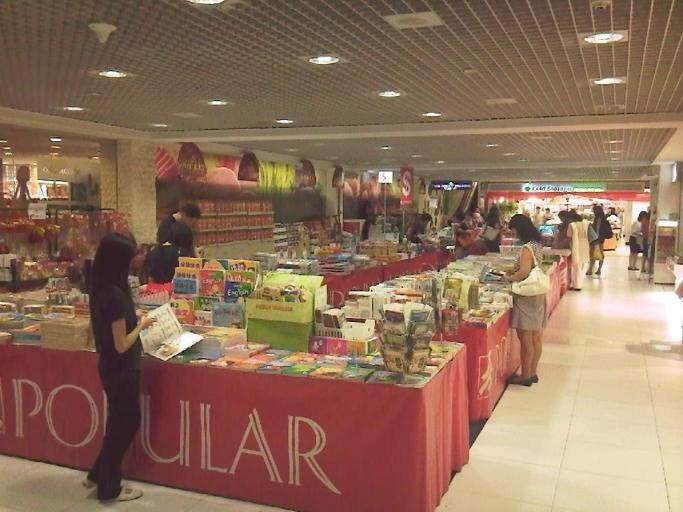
[599,219,613,239]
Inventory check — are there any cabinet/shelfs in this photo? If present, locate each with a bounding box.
[181,200,274,260]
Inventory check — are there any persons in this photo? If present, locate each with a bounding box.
[157,206,203,259]
[145,221,194,283]
[627,211,647,270]
[83,232,156,503]
[405,205,621,291]
[504,215,550,384]
[642,210,651,272]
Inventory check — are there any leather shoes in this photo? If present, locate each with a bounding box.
[530,374,538,383]
[509,376,531,387]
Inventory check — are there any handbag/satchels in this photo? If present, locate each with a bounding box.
[511,244,550,297]
[479,224,500,241]
[458,229,480,247]
[587,224,598,242]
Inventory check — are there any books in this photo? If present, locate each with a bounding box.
[127,239,503,382]
[0,239,98,350]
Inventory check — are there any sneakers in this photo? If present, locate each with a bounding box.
[111,486,143,502]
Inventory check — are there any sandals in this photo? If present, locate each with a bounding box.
[628,267,631,270]
[632,266,639,270]
